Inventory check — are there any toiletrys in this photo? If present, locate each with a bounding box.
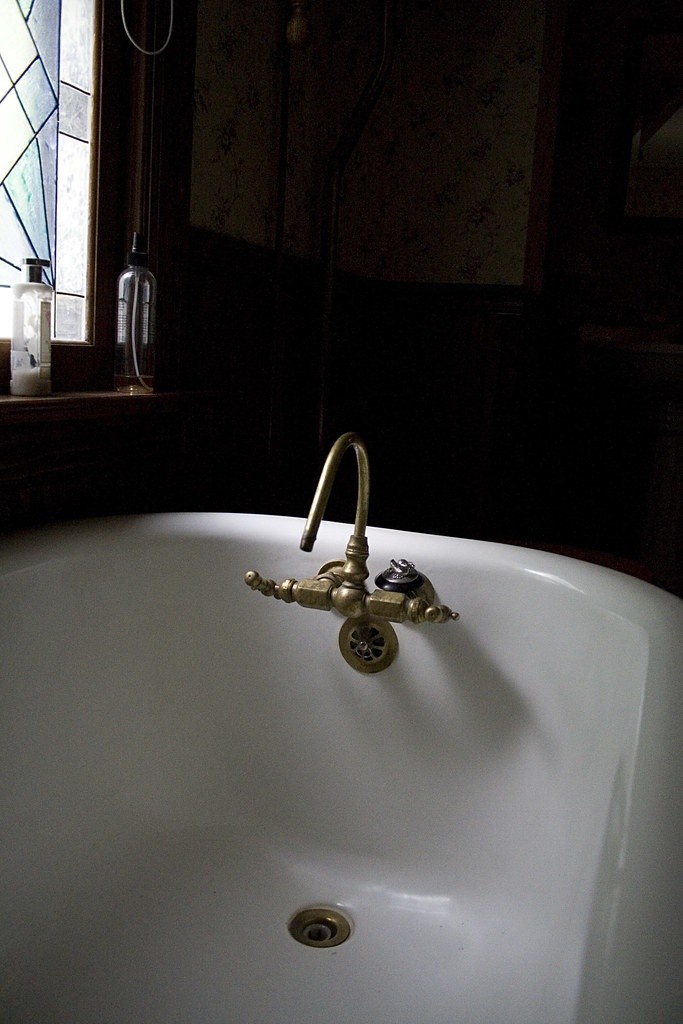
[113,232,157,395]
[10,259,52,396]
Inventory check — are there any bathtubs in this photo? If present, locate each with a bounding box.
[1,513,681,1024]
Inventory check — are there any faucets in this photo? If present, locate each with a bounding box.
[244,432,369,619]
